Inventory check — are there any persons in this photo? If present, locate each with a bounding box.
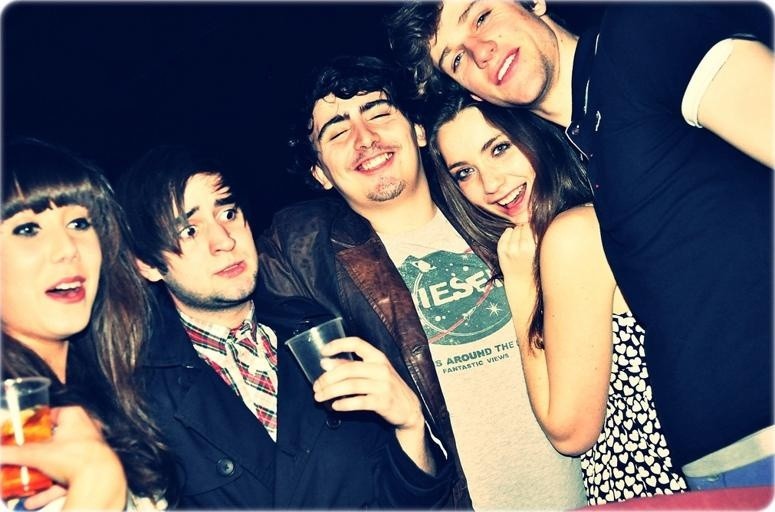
[79,143,458,512]
[422,83,685,506]
[384,0,774,492]
[1,136,166,512]
[252,57,592,512]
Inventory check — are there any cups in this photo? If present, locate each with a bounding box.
[285,316,353,385]
[0,376,53,501]
